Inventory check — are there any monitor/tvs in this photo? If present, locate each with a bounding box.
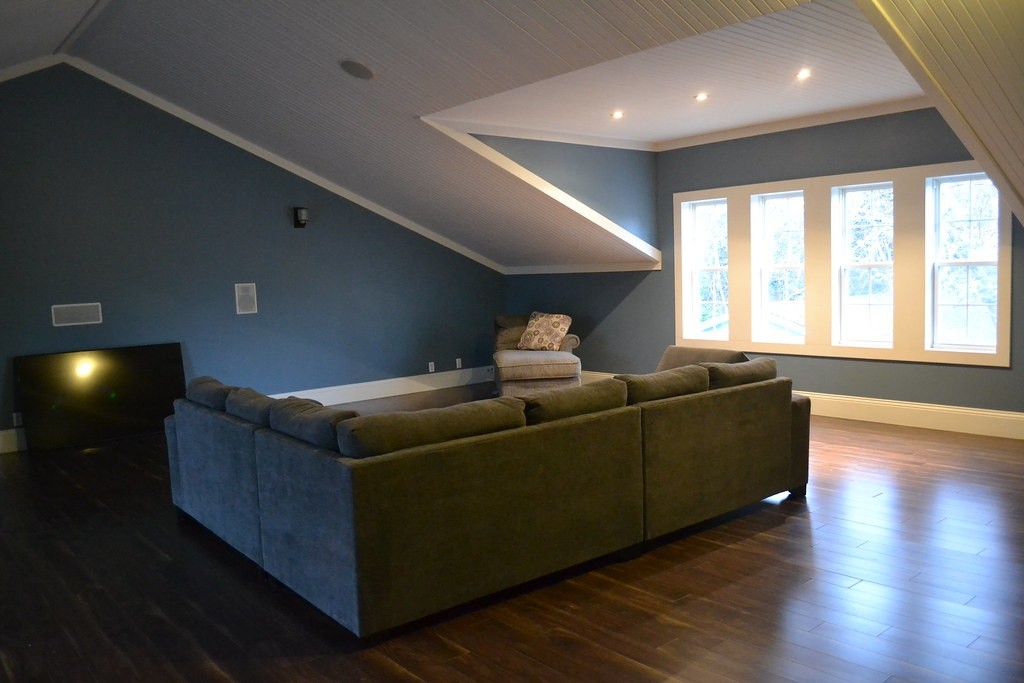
[15,343,187,452]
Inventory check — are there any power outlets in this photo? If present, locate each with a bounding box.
[456,358,462,369]
[429,362,435,372]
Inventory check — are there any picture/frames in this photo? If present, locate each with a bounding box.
[235,283,258,314]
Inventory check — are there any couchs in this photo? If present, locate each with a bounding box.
[160,374,278,571]
[614,357,811,550]
[657,346,750,374]
[252,379,647,642]
[492,333,582,397]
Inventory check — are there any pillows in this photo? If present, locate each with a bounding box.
[517,311,572,351]
[494,315,530,350]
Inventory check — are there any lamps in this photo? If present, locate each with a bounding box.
[293,206,310,228]
